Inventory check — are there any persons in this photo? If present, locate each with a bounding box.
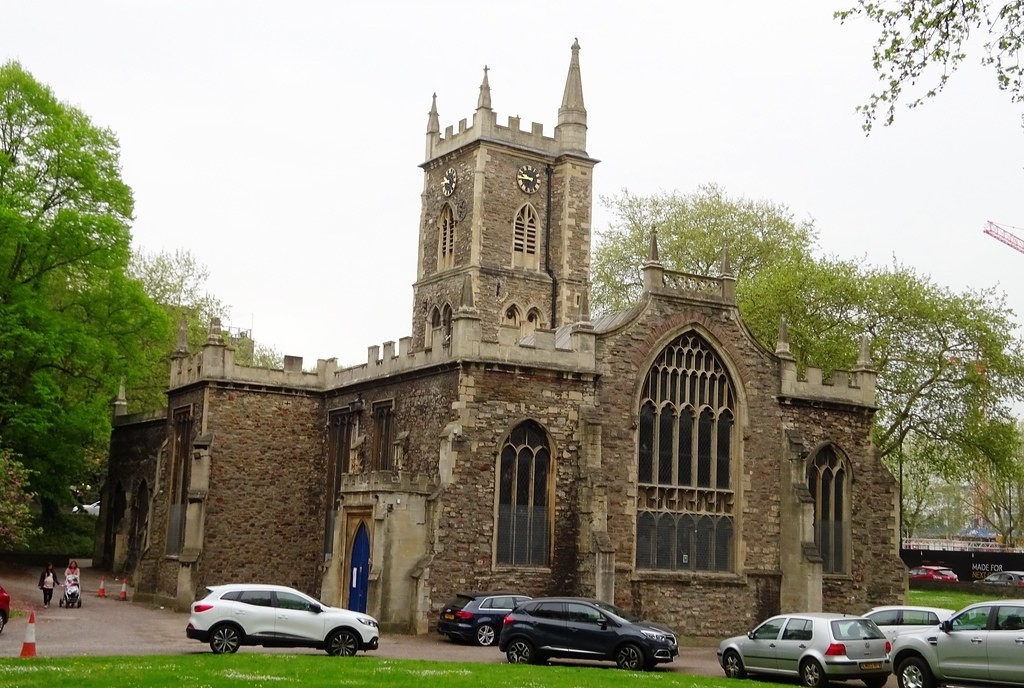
[61,561,79,607]
[38,563,61,608]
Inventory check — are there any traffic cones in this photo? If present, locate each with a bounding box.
[16,609,38,660]
[95,575,108,597]
[113,578,132,602]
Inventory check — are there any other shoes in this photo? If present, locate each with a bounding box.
[48,601,50,605]
[44,605,47,609]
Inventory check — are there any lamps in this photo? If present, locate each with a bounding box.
[193,452,212,461]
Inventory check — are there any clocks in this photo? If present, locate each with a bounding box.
[440,168,457,197]
[517,164,542,194]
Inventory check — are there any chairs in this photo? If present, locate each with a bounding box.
[1006,616,1021,629]
[847,624,861,639]
[777,628,789,638]
[575,607,590,623]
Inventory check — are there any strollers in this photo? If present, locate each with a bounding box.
[58,574,84,609]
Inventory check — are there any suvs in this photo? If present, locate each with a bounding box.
[185,582,380,658]
[887,599,1023,688]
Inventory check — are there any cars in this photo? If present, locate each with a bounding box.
[437,592,534,647]
[718,612,893,688]
[908,566,959,585]
[0,587,10,633]
[973,571,1024,588]
[839,605,966,655]
[500,596,681,671]
